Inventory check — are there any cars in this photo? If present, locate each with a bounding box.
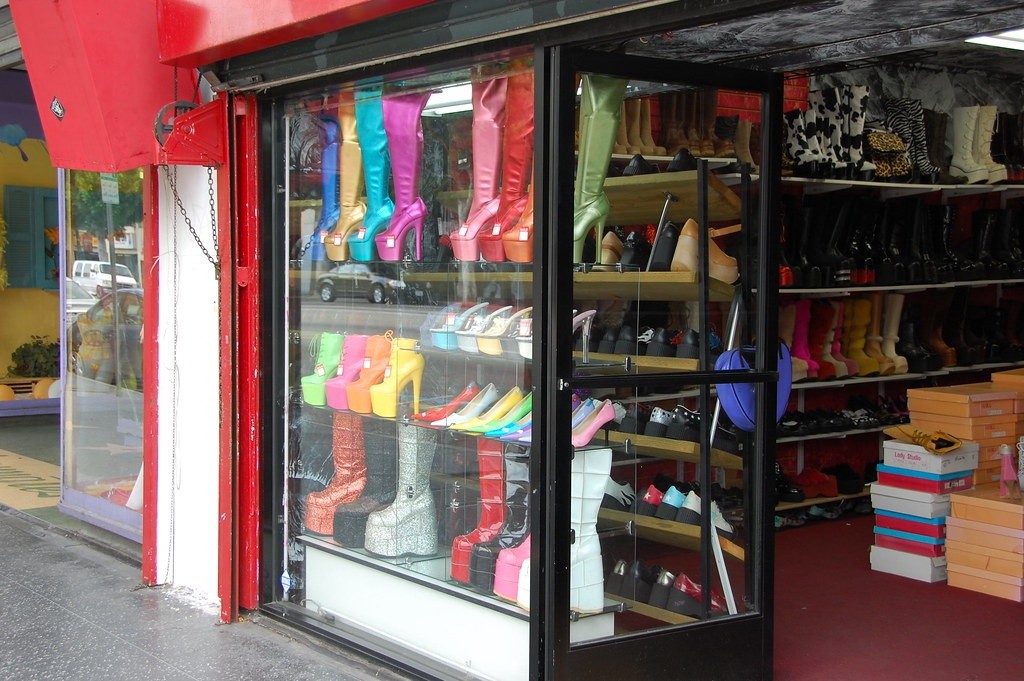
[316,261,430,306]
[65,276,144,393]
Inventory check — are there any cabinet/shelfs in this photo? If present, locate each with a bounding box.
[287,50,741,681]
[604,157,738,468]
[718,172,1024,514]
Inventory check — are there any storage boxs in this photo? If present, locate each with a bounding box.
[869,367,1024,603]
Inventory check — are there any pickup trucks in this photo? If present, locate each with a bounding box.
[289,259,325,297]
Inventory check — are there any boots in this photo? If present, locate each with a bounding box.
[287,69,1024,620]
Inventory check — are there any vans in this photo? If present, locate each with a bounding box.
[73,260,137,299]
